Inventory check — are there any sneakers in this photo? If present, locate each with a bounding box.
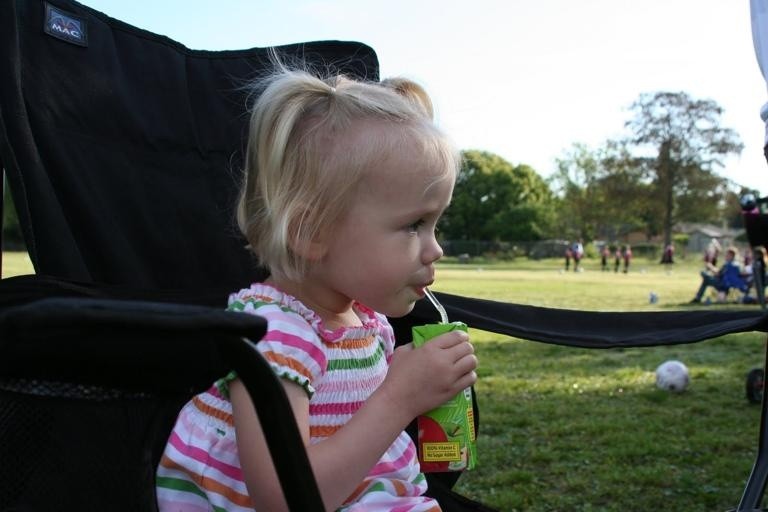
[689,299,703,305]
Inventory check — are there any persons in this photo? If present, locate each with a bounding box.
[666,242,674,262]
[153,68,479,512]
[598,243,632,273]
[690,238,767,305]
[563,238,586,272]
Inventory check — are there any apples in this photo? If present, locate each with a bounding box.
[438,421,466,449]
[418,416,449,472]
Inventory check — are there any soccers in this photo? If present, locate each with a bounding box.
[656,361,689,393]
